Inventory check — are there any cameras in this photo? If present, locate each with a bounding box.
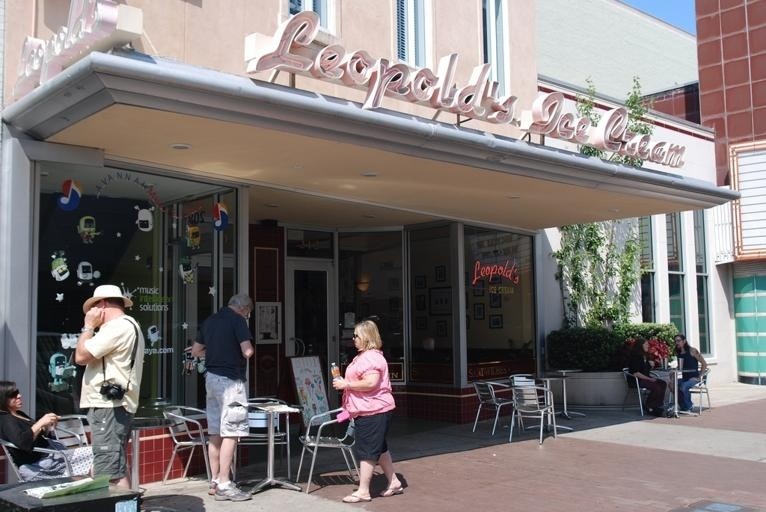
[100,382,125,400]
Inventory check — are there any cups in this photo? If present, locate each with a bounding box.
[47,421,55,432]
[649,357,668,371]
[678,357,684,371]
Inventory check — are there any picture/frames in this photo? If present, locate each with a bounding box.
[379,262,503,339]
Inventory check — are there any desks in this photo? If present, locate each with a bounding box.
[0,475,141,512]
[129,416,177,512]
[651,369,697,418]
[243,407,301,495]
[549,367,587,421]
[520,374,574,432]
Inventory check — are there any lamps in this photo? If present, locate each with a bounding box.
[355,282,369,293]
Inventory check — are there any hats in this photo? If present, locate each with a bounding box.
[83,285,133,314]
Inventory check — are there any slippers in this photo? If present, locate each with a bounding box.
[380,487,404,496]
[343,495,371,503]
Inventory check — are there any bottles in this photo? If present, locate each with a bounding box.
[330,361,342,381]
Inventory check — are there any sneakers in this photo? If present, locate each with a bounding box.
[215,482,252,501]
[208,480,242,494]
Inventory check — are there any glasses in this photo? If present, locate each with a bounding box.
[354,334,358,338]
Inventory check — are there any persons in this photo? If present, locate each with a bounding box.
[191,293,255,502]
[74,284,146,495]
[0,380,73,481]
[626,336,668,417]
[667,334,708,416]
[332,320,404,503]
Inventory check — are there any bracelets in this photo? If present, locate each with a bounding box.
[80,327,95,334]
[345,382,351,391]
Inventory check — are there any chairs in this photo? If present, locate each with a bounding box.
[162,404,234,485]
[47,414,91,450]
[231,397,291,486]
[294,407,359,495]
[507,373,550,433]
[507,385,557,445]
[471,381,524,436]
[621,367,661,417]
[0,438,72,484]
[688,366,713,417]
